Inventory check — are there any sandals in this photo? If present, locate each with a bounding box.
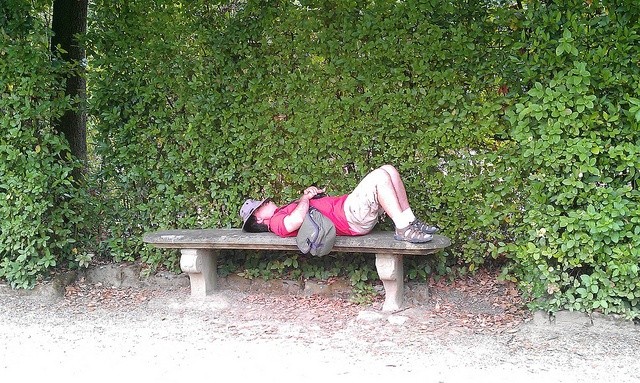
[394,224,433,242]
[409,217,439,233]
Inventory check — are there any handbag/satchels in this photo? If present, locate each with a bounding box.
[296,205,336,256]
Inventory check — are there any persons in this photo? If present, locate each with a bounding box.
[239,165,440,244]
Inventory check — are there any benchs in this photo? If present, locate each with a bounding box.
[144,230,449,313]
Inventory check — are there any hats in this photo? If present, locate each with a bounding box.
[240,196,278,231]
